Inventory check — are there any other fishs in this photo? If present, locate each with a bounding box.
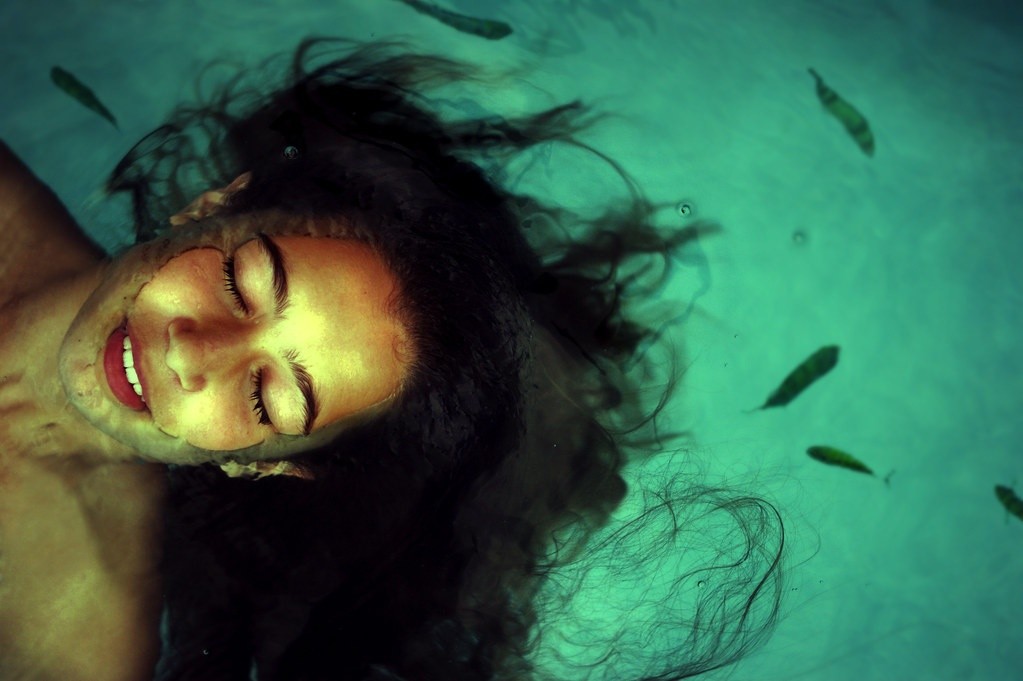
[50,64,122,134]
[806,65,877,160]
[992,481,1023,523]
[402,1,513,42]
[805,446,897,492]
[754,342,842,414]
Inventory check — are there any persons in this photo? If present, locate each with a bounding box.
[1,30,822,680]
